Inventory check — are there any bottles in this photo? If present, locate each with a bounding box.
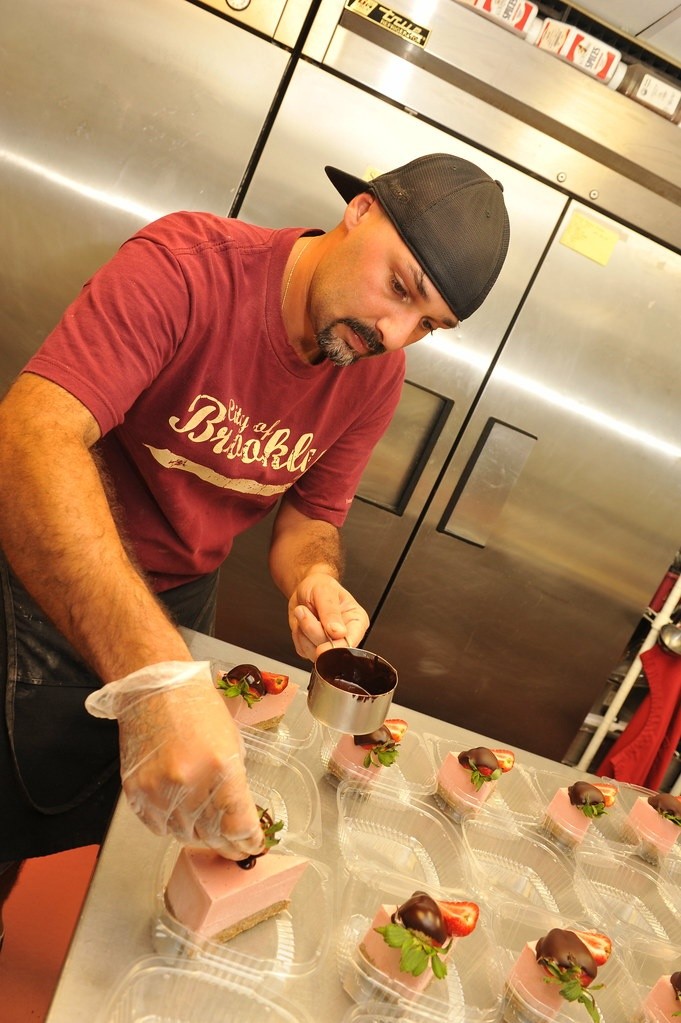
[534,16,627,91]
[453,0,543,44]
[605,62,681,123]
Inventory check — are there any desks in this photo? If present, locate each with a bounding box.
[42,624,681,1023]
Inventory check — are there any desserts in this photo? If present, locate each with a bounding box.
[162,663,681,1023]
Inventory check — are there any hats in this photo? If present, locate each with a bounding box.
[325,154,510,324]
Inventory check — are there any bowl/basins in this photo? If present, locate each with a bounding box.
[99,661,681,1023]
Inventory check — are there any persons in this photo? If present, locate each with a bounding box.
[0,152,510,908]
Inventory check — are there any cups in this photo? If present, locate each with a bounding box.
[308,603,399,736]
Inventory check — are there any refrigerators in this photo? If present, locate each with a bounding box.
[231,1,681,764]
[2,1,318,401]
